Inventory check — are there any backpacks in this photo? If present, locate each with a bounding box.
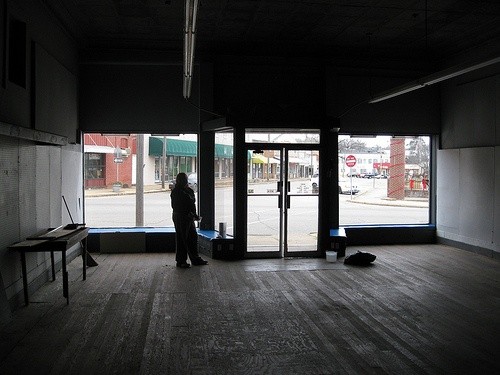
[344,250,377,266]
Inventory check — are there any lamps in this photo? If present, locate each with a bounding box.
[367,52,500,103]
[182,0,199,98]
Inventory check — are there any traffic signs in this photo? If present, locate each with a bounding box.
[113,158,124,164]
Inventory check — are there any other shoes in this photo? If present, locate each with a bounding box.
[199,259,208,265]
[177,261,190,268]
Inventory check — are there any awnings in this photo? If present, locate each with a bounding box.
[252,153,311,168]
[147,136,252,161]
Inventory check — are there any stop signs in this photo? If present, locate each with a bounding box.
[345,155,357,167]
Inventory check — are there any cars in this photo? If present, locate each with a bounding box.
[168,172,198,194]
[311,175,359,195]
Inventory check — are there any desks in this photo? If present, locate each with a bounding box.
[4,227,89,307]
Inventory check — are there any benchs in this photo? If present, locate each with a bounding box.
[329,225,433,257]
[87,228,234,260]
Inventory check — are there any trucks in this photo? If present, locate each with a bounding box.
[347,168,375,179]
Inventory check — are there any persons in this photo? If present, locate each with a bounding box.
[170,172,208,268]
